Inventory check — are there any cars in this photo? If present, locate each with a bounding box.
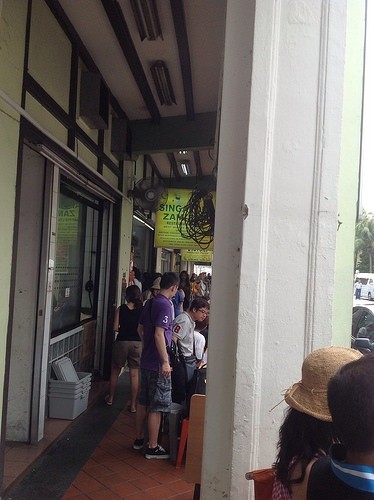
[352,306,374,357]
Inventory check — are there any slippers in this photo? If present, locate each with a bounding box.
[127,399,137,413]
[104,394,114,405]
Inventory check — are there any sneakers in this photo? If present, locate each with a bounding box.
[144,445,171,460]
[131,437,145,451]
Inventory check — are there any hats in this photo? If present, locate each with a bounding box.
[269,344,365,424]
[150,277,161,289]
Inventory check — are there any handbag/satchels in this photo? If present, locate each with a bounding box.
[244,468,276,500]
[165,340,179,368]
[181,353,198,381]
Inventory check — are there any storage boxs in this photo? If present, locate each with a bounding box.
[48,357,92,421]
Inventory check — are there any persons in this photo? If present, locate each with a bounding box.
[307,351,374,500]
[272,346,365,500]
[130,267,212,441]
[133,271,180,459]
[105,284,143,413]
[355,279,362,299]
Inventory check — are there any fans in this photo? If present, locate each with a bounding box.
[127,177,169,212]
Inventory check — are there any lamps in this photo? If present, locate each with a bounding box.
[150,61,178,108]
[179,161,192,176]
[131,0,165,43]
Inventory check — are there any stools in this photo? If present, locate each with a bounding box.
[163,403,189,469]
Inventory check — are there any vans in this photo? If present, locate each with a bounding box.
[354,273,374,300]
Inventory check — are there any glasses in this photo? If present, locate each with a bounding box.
[199,309,210,316]
